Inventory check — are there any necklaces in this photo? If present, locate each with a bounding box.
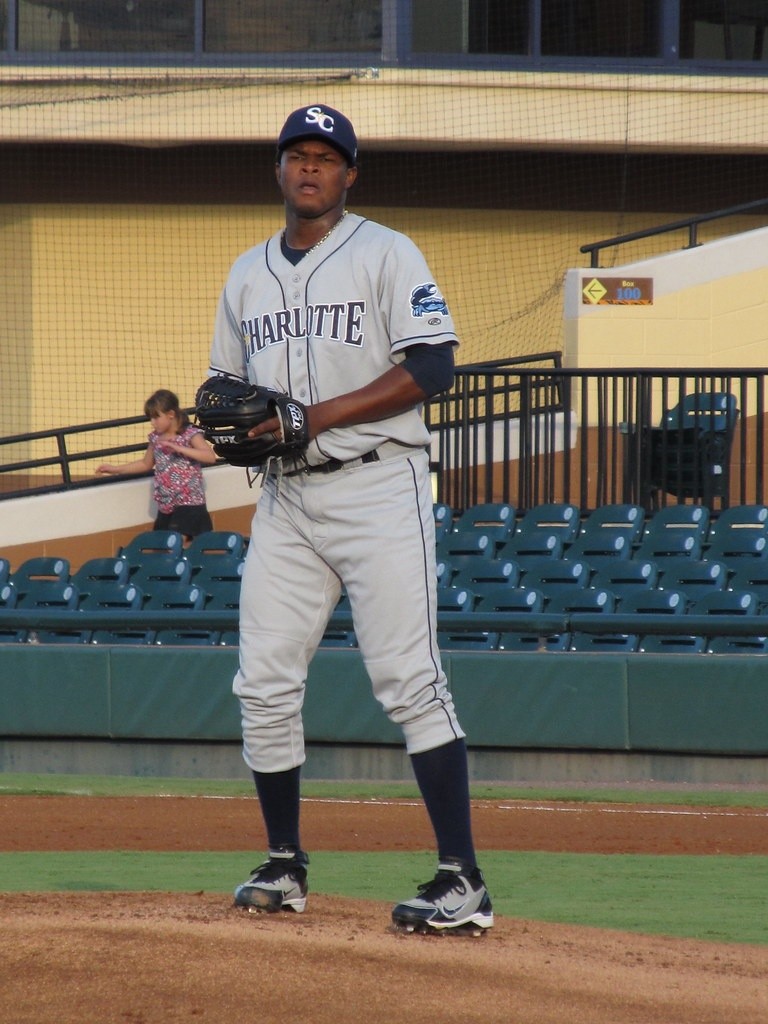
[306,209,348,256]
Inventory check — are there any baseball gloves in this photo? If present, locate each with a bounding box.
[192,364,312,498]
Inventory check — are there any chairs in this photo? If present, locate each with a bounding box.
[0,393,768,653]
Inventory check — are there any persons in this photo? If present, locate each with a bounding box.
[195,104,495,937]
[94,389,216,554]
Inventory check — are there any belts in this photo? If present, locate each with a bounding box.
[272,448,379,478]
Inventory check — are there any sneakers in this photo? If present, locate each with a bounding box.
[233,845,310,914]
[391,858,493,938]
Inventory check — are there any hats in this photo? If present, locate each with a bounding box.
[276,104,357,169]
